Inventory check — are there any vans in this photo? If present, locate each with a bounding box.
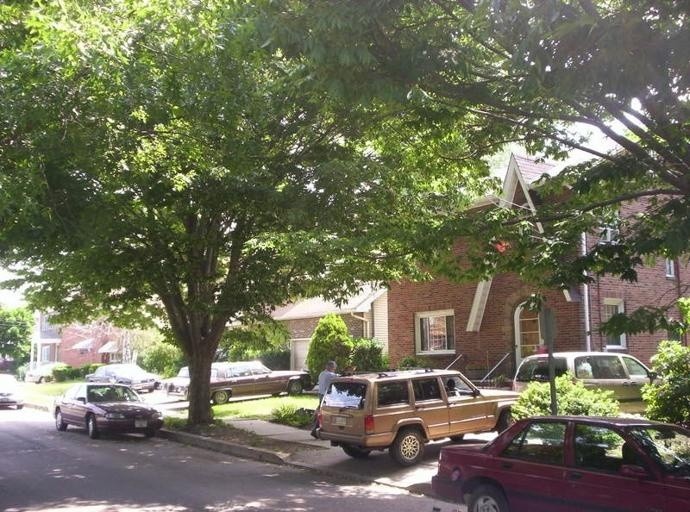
[512,352,661,415]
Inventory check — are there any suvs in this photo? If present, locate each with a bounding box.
[315,367,524,467]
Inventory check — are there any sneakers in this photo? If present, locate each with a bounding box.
[311,431,319,439]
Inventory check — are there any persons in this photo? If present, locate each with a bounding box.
[310,360,354,439]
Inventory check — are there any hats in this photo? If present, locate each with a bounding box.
[326,361,337,368]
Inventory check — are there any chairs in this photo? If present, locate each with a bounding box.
[622,441,644,467]
[580,445,607,468]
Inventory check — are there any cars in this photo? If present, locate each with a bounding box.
[84,363,162,394]
[431,416,690,512]
[0,373,25,408]
[161,361,312,405]
[53,382,164,438]
[25,363,67,383]
